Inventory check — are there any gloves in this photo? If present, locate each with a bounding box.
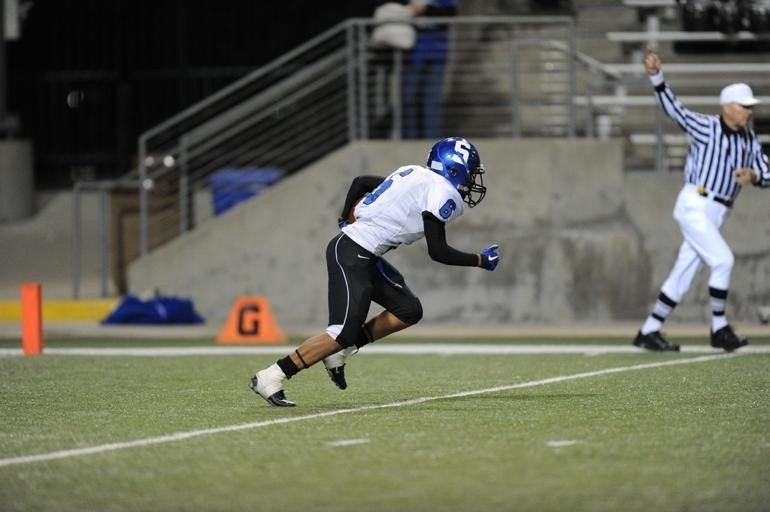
[478,241,501,272]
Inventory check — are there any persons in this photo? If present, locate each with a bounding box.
[631,54,770,351]
[246,135,501,408]
[398,0,474,141]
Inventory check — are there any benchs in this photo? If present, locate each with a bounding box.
[575,28,769,169]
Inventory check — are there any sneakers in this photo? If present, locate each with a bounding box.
[632,330,680,353]
[710,324,747,352]
[246,375,295,410]
[321,348,349,391]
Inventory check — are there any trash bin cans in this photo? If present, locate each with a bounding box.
[211,166,285,216]
[0,118,36,223]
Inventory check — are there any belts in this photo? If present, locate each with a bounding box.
[698,188,736,211]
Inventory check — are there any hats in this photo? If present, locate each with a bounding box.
[719,81,761,107]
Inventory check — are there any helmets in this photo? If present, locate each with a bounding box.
[427,136,488,209]
